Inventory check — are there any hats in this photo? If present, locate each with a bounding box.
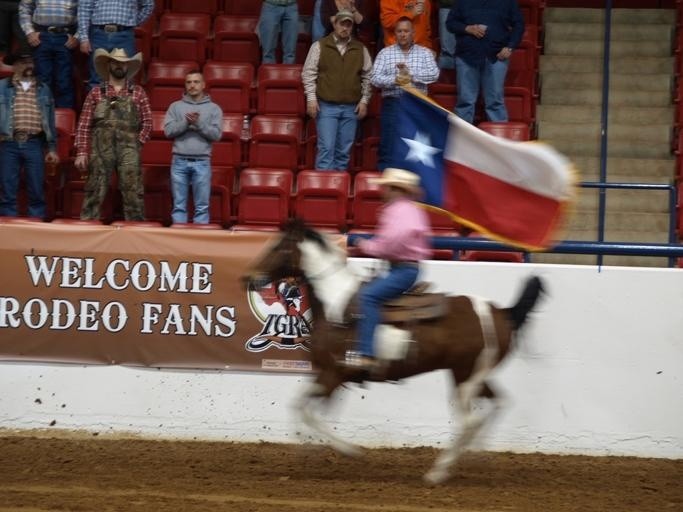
[367,168,421,195]
[4,46,34,65]
[93,48,143,80]
[335,9,353,24]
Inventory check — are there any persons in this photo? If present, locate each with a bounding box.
[1,0,156,228]
[260,1,458,68]
[302,10,373,170]
[371,17,439,169]
[329,163,427,368]
[446,1,523,124]
[160,71,224,223]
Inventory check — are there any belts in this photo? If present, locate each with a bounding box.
[14,132,43,142]
[91,24,135,31]
[33,22,78,33]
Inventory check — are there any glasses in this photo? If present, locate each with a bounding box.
[111,97,117,109]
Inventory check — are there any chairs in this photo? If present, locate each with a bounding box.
[50,3,540,171]
[295,169,351,230]
[234,170,292,228]
[416,206,526,264]
[352,172,388,228]
[51,172,233,232]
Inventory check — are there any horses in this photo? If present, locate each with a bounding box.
[238,214,544,487]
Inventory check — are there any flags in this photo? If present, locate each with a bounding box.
[393,86,573,255]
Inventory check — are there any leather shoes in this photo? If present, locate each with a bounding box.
[338,357,376,367]
